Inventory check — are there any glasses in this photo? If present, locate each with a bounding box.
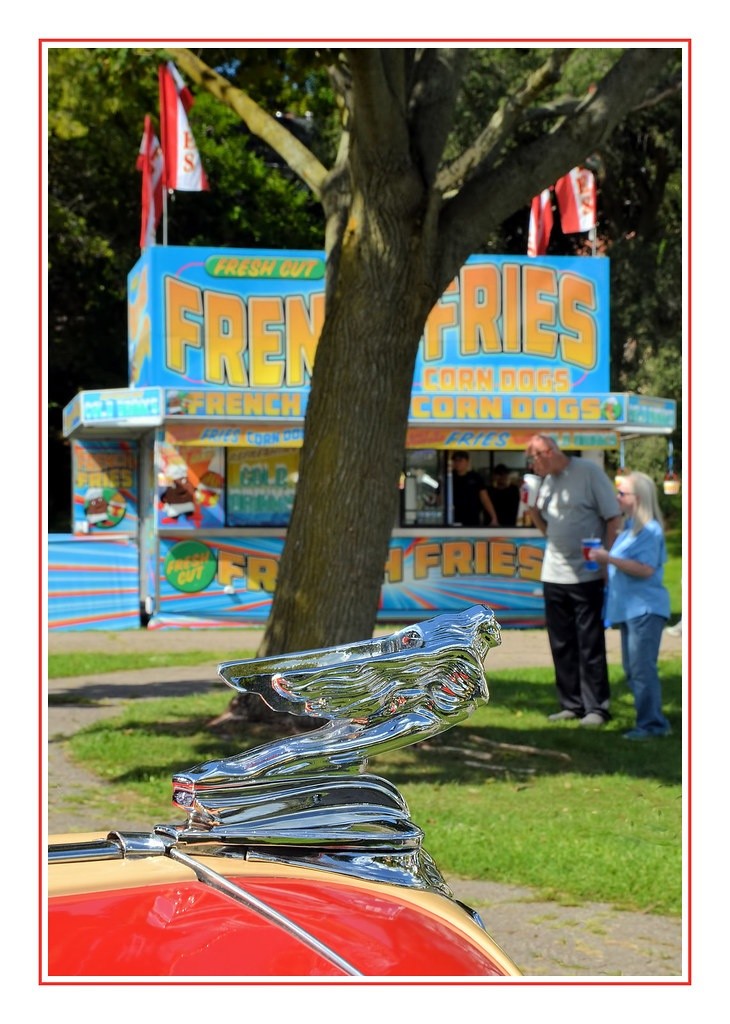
[527,448,553,464]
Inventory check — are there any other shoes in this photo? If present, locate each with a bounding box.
[580,713,604,727]
[623,725,673,740]
[548,710,575,721]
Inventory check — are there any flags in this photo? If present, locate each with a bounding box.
[137,114,167,248]
[527,189,552,258]
[554,168,596,233]
[159,60,209,192]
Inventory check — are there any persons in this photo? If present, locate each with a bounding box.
[526,435,623,728]
[588,472,673,741]
[483,463,520,530]
[450,451,501,529]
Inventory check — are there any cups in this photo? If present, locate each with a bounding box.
[581,538,601,572]
[523,474,542,508]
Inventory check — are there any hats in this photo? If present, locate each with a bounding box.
[494,464,512,474]
[451,451,469,461]
